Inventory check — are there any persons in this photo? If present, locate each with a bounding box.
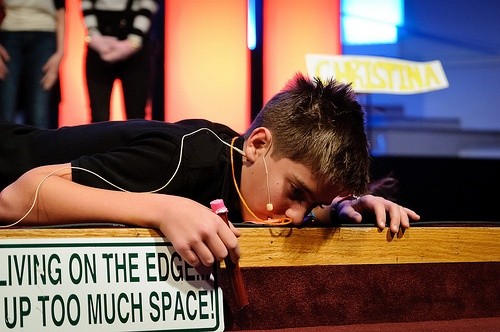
[81,0,158,124]
[0,70,420,267]
[0,0,66,129]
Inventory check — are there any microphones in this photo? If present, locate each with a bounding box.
[262,136,273,211]
